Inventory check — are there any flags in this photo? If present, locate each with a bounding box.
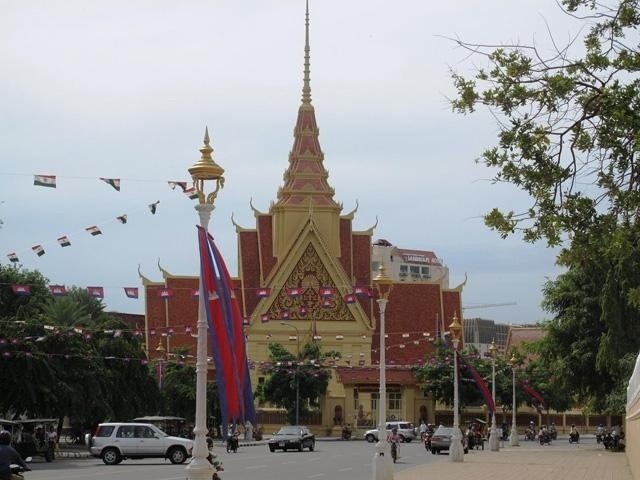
[451,347,499,419]
[115,214,129,226]
[32,173,56,189]
[99,177,121,193]
[195,224,261,443]
[513,374,547,406]
[57,236,71,250]
[168,180,188,193]
[2,280,517,387]
[7,251,19,264]
[183,186,201,201]
[85,225,103,238]
[147,200,162,215]
[31,245,46,258]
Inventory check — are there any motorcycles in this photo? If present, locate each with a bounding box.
[424,431,433,451]
[9,456,32,480]
[524,425,624,449]
[227,431,239,453]
[342,428,352,440]
[387,440,401,464]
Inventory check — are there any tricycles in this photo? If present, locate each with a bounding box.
[0,418,58,463]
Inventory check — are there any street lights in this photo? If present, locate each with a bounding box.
[509,353,519,445]
[488,336,500,452]
[280,322,300,425]
[449,308,463,462]
[370,256,395,480]
[156,335,167,389]
[185,125,225,480]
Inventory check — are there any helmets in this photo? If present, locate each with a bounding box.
[0,430,10,445]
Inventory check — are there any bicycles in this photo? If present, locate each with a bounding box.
[65,432,82,445]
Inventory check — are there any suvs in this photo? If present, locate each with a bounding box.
[363,421,420,443]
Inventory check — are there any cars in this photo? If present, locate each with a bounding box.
[431,428,469,454]
[267,425,315,452]
[84,422,194,466]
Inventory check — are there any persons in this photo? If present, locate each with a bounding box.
[187,438,223,476]
[417,413,626,460]
[345,423,352,432]
[142,421,262,451]
[22,425,58,449]
[0,430,32,480]
[388,427,401,449]
